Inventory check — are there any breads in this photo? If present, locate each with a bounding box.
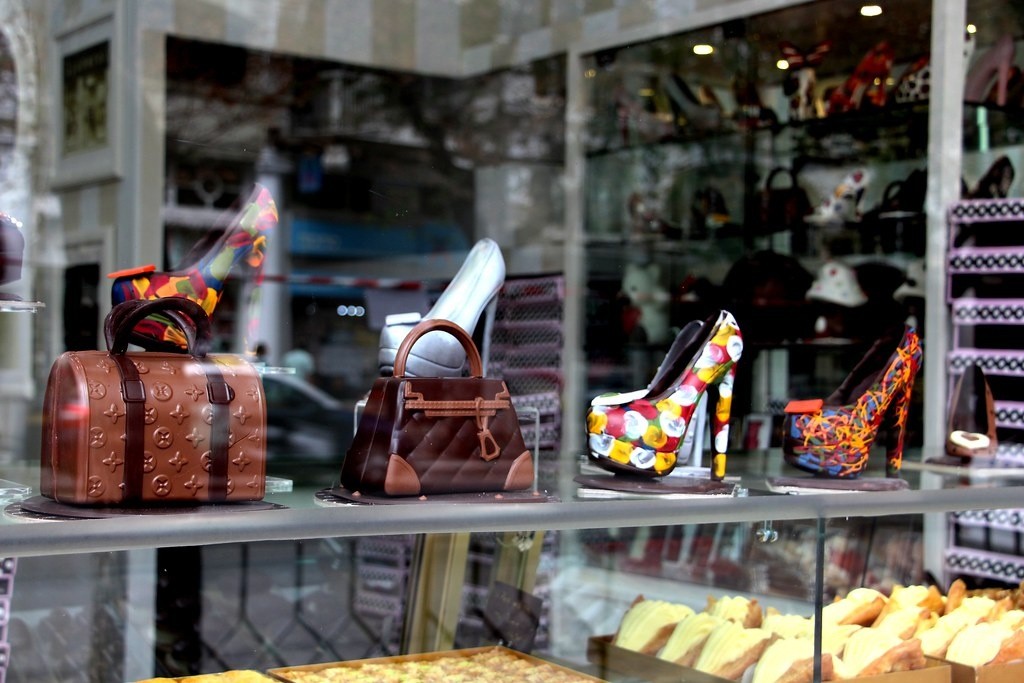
[127,650,608,683]
[613,573,1024,683]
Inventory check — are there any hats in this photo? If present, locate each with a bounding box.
[804,260,867,308]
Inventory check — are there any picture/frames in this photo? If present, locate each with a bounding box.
[50,225,114,363]
[45,3,126,191]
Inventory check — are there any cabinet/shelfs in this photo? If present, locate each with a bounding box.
[135,0,1024,461]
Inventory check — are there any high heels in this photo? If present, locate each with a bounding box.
[944,364,998,461]
[584,309,744,482]
[786,325,922,477]
[584,29,1016,319]
[377,239,508,381]
[112,182,280,358]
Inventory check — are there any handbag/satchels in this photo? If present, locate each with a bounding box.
[763,166,815,226]
[38,299,267,507]
[339,322,536,496]
